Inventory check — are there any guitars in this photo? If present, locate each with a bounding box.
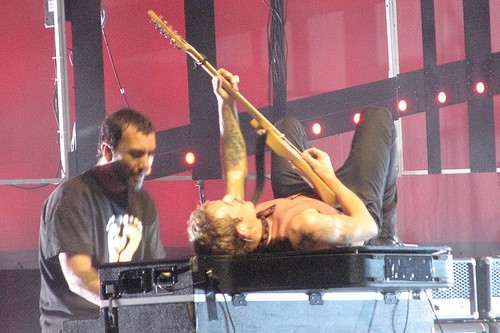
[146,8,345,211]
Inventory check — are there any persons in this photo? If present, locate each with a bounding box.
[187,68,417,257]
[35,107,173,332]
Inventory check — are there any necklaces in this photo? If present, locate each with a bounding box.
[253,214,270,252]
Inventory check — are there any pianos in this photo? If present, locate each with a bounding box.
[60,242,456,333]
